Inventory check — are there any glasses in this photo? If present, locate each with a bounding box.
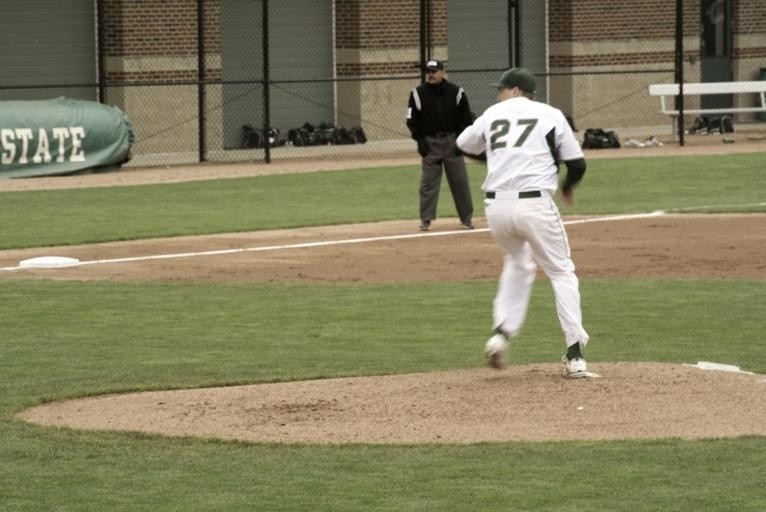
[425,69,438,74]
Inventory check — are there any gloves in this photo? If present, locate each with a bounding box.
[419,137,431,157]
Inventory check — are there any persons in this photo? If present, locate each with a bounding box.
[406,60,476,230]
[453,68,590,379]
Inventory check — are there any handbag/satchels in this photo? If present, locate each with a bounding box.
[243,122,367,150]
[690,116,732,135]
[581,128,621,149]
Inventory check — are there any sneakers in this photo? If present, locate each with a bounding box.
[482,334,509,370]
[562,353,589,380]
[462,220,476,229]
[418,220,432,232]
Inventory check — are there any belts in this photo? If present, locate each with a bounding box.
[426,131,453,138]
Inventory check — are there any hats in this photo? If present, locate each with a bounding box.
[423,60,444,71]
[487,67,537,95]
